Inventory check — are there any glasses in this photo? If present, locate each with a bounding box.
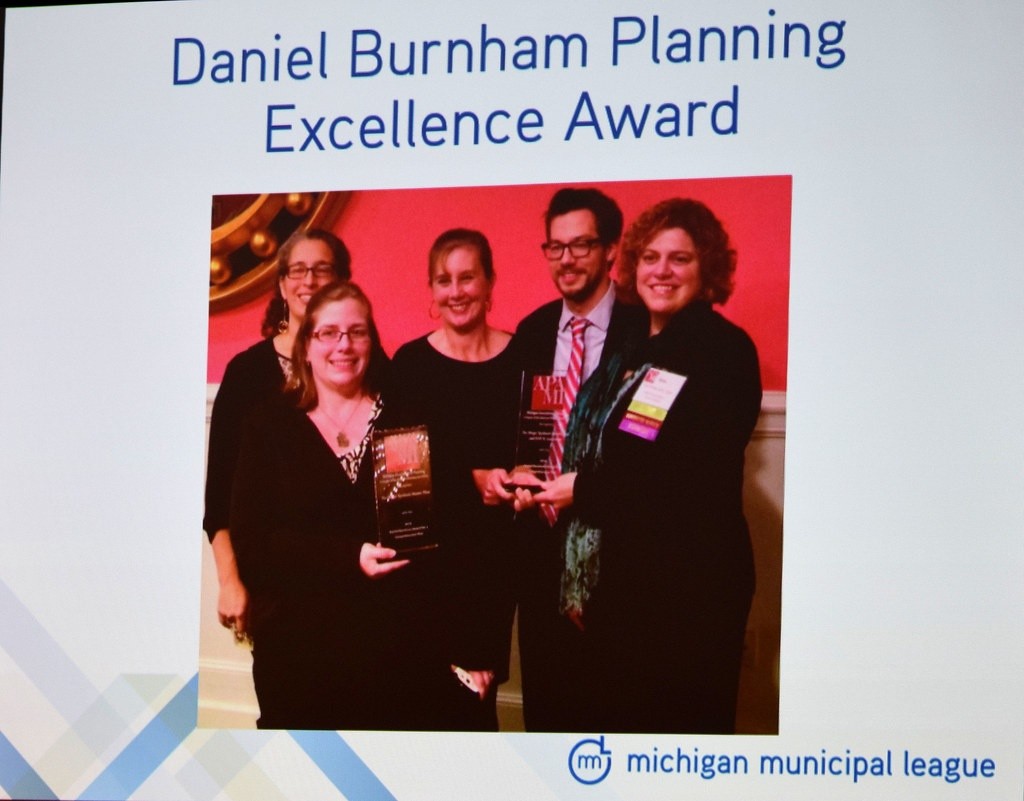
[305,323,372,348]
[539,235,599,261]
[276,258,335,282]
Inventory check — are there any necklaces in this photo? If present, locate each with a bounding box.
[315,387,365,448]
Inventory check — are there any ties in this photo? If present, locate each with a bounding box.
[534,316,599,528]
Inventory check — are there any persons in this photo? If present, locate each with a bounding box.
[379,222,533,734]
[202,226,396,733]
[522,193,764,739]
[501,186,639,735]
[227,274,449,730]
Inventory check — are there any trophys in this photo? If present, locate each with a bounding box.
[500,359,583,505]
[364,423,448,563]
